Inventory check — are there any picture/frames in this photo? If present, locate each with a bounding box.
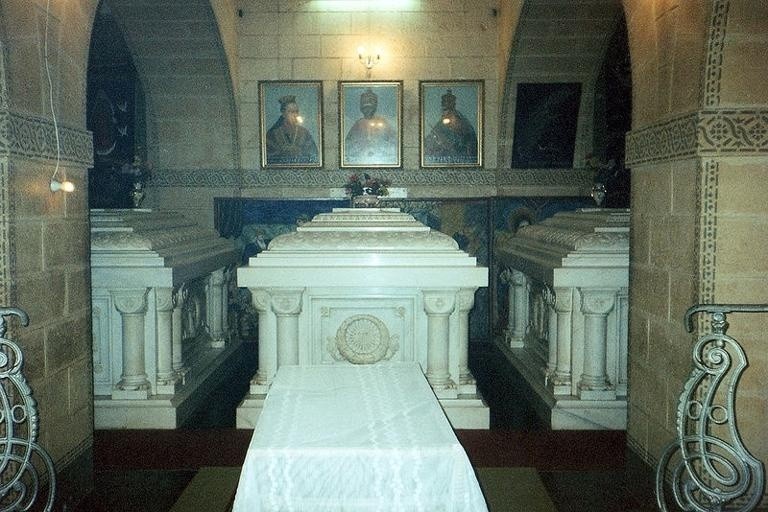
[257,80,324,169]
[418,79,485,169]
[338,80,403,170]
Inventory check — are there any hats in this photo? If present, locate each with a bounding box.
[278,95,296,104]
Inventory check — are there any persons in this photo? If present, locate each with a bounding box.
[424,92,477,162]
[262,95,320,166]
[344,92,397,161]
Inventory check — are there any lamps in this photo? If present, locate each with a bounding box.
[356,43,384,70]
[50,179,74,193]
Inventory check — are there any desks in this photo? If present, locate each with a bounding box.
[248,361,463,512]
